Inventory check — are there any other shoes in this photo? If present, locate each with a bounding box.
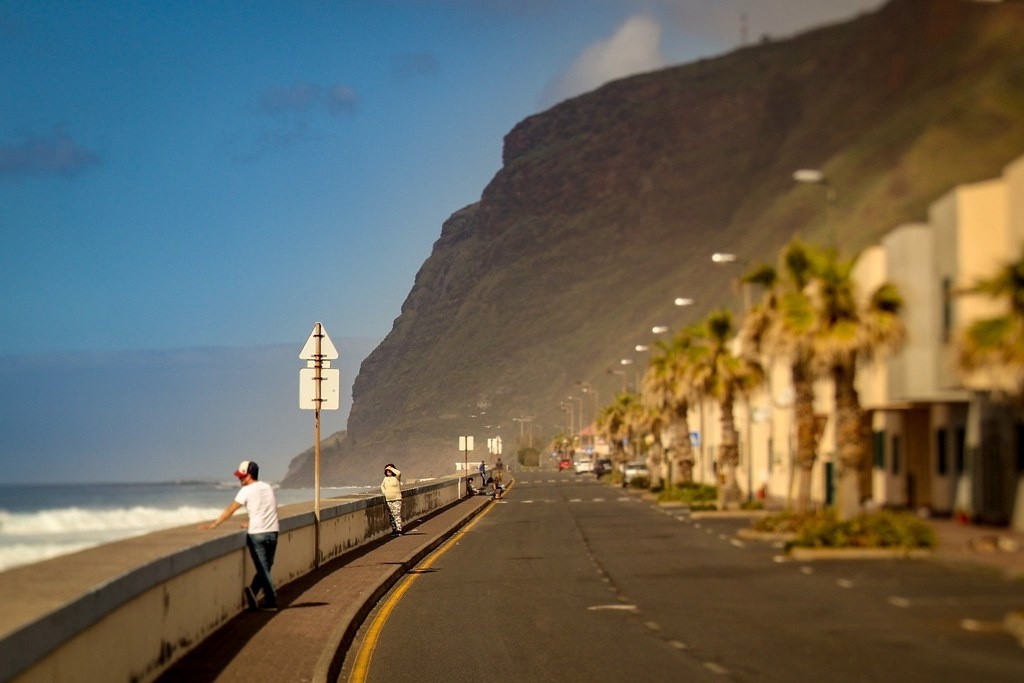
[258,602,278,612]
[246,587,259,610]
[391,531,396,536]
[396,531,402,537]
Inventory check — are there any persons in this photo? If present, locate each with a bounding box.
[495,476,508,492]
[199,461,280,611]
[381,464,403,537]
[479,461,487,487]
[486,477,503,499]
[495,458,504,481]
[467,478,486,495]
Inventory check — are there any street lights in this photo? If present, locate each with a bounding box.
[567,396,582,451]
[583,389,599,417]
[560,402,573,438]
[513,418,532,449]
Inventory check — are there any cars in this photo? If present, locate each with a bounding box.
[622,462,651,488]
[560,460,571,470]
[574,457,593,473]
[595,459,612,473]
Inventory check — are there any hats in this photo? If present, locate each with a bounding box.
[233,460,258,477]
